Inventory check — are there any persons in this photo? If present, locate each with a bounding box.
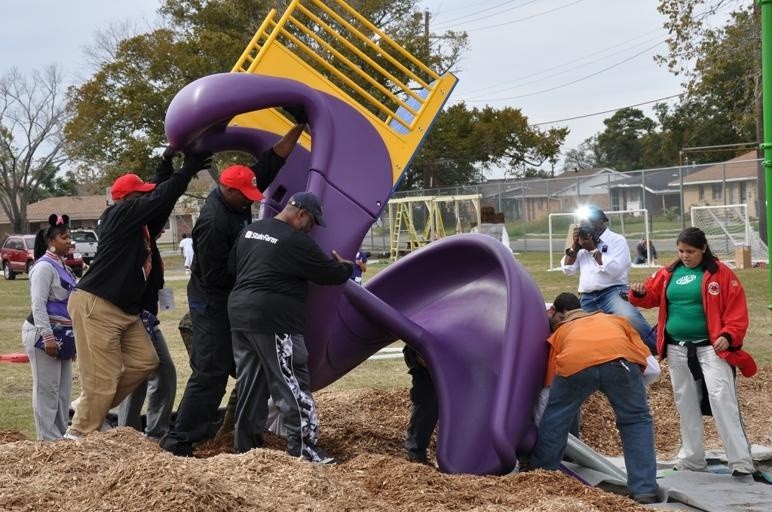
[21,105,368,466]
[531,205,758,502]
[402,344,439,464]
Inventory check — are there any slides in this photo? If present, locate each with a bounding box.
[166,73,548,476]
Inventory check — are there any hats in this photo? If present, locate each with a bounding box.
[286,191,326,228]
[112,173,157,201]
[219,164,265,201]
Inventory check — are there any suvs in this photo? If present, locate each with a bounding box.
[0,233,84,284]
[64,227,102,268]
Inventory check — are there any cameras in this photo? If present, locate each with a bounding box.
[575,207,602,245]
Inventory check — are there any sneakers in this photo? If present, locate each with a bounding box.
[321,457,338,465]
[672,463,691,471]
[731,466,751,476]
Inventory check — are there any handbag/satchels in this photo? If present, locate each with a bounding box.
[34,322,76,361]
